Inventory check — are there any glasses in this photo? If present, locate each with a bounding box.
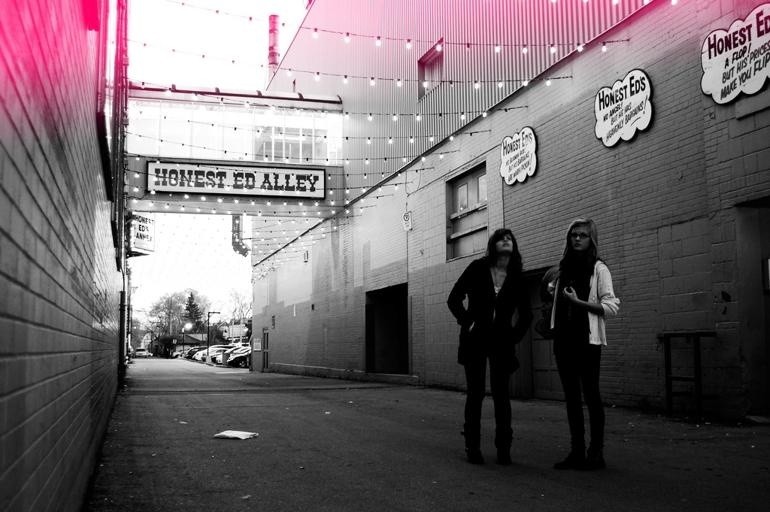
[572,233,590,238]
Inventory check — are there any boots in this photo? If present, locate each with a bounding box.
[463,424,486,466]
[553,441,606,472]
[495,428,514,466]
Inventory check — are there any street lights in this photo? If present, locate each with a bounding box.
[207,311,221,363]
[181,330,185,355]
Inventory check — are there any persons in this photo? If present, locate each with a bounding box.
[547,218,620,470]
[447,228,534,464]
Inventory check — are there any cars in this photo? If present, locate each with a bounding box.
[172,343,251,368]
[129,346,154,358]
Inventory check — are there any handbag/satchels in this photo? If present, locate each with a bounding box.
[534,318,551,341]
